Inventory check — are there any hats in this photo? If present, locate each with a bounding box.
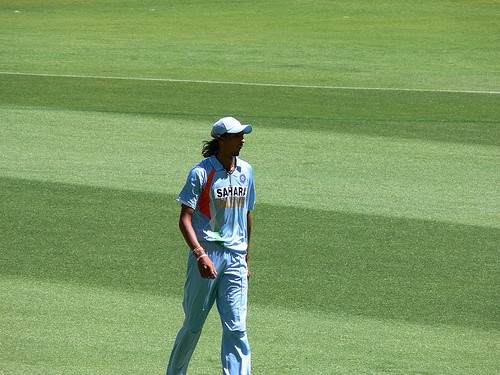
[210,117,253,138]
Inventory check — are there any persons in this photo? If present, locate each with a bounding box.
[165,116,256,375]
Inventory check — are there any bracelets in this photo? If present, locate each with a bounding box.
[191,246,205,255]
[197,254,208,262]
